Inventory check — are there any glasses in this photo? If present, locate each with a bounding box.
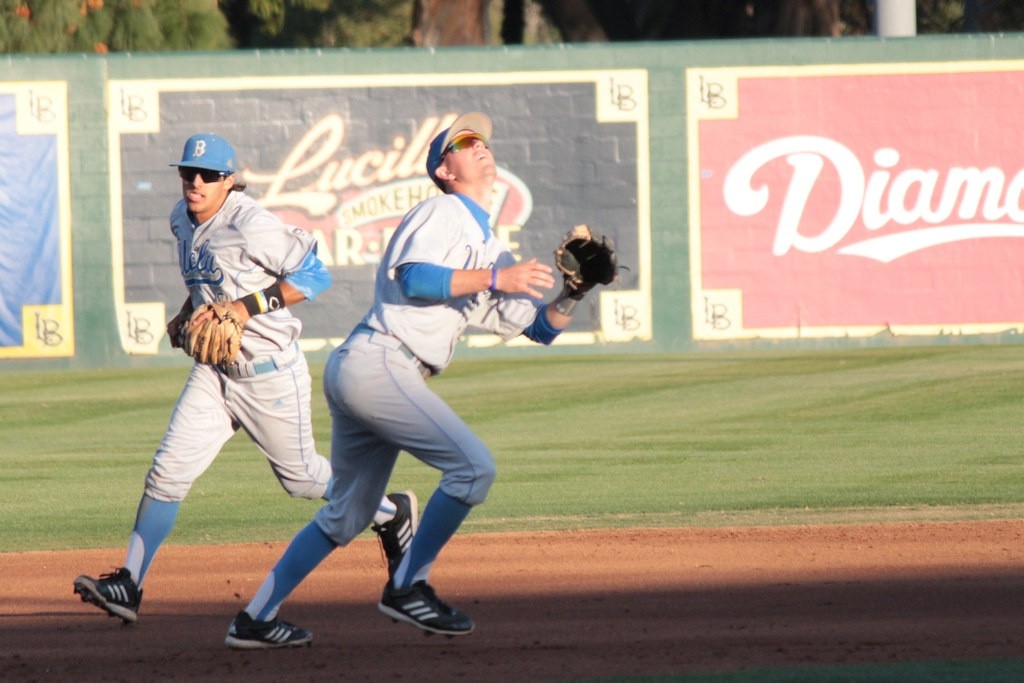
[440,133,489,161]
[178,166,230,183]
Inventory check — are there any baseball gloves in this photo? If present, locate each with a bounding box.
[172,292,250,371]
[550,226,622,304]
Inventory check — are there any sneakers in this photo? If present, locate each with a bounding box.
[224,611,313,650]
[371,490,419,578]
[378,580,475,638]
[74,565,143,624]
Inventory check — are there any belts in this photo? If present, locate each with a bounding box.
[215,341,300,379]
[352,323,434,380]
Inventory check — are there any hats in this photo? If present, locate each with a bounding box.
[169,132,237,172]
[426,112,493,181]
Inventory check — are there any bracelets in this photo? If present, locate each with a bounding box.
[490,268,498,292]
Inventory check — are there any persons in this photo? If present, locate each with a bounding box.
[72,134,417,625]
[222,112,592,652]
